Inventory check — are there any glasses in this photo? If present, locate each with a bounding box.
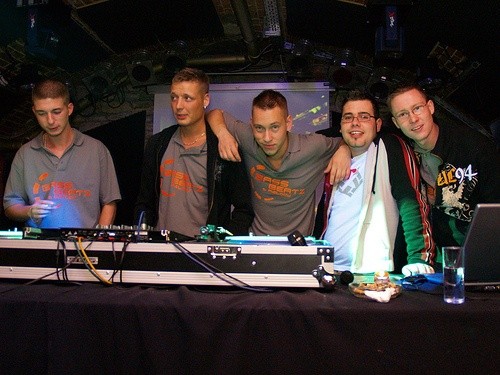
[341,113,377,123]
[395,102,427,122]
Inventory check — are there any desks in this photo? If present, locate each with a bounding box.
[0,283,500,375]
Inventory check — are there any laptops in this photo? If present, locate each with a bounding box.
[425,204,500,291]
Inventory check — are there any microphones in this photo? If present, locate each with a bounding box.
[288,230,308,246]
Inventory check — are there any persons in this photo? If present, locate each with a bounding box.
[3,80,122,229]
[139,68,251,237]
[386,83,500,274]
[317,90,436,276]
[207,90,351,237]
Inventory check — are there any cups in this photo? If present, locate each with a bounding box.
[442,247,466,304]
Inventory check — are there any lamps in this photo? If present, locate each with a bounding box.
[287,40,394,100]
[90,60,122,94]
[161,40,189,76]
[131,49,154,83]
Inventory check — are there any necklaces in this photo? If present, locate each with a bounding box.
[190,131,205,146]
